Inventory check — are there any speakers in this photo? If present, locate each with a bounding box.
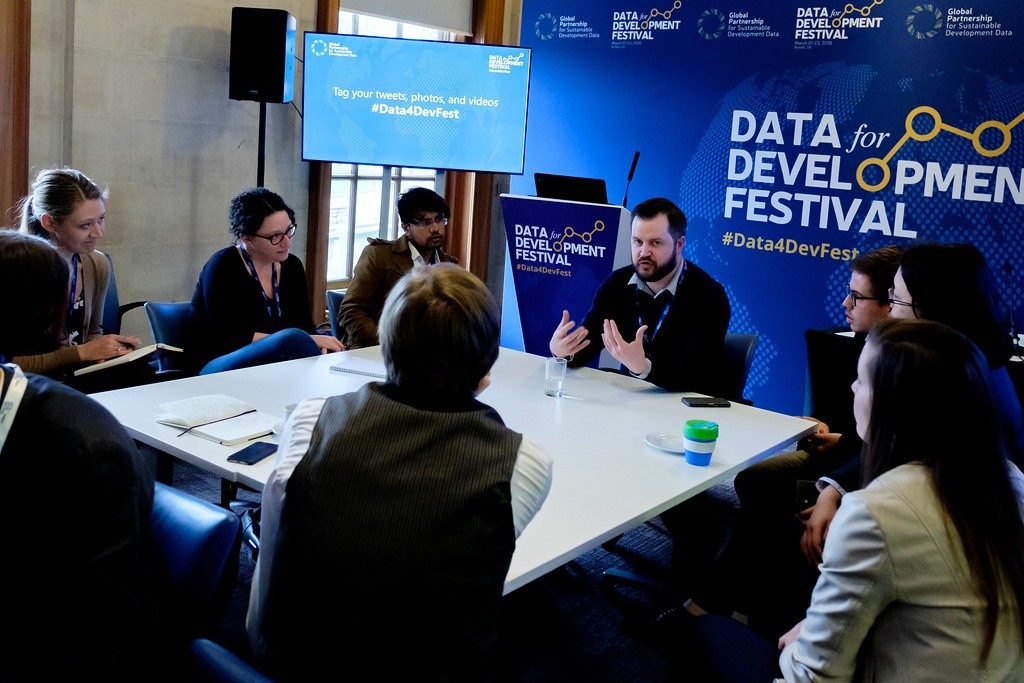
[229,7,297,104]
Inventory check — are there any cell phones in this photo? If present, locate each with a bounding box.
[227,441,279,466]
[681,397,731,409]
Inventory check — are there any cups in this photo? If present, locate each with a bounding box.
[683,419,718,466]
[284,404,298,425]
[544,357,567,396]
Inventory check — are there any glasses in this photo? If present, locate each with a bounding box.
[888,285,918,308]
[407,217,448,228]
[846,285,881,306]
[248,223,297,245]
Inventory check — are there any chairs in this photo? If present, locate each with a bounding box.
[150,285,733,683]
[804,328,862,449]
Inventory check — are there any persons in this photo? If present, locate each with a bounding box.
[734,244,905,510]
[549,198,731,400]
[0,167,173,487]
[246,261,552,683]
[659,317,1024,683]
[0,363,154,683]
[618,243,1024,641]
[174,186,346,378]
[337,187,459,351]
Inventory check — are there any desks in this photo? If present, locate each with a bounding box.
[75,328,817,602]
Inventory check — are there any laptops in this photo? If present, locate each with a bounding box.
[534,173,617,206]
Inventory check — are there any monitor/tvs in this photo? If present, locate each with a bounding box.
[300,31,532,175]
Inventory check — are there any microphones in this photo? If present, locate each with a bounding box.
[622,151,641,208]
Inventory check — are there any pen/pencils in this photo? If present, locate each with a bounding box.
[98,324,134,347]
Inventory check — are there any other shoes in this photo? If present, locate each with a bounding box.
[643,598,699,626]
[781,588,809,624]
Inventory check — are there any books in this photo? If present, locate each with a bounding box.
[155,394,283,445]
[74,343,183,377]
[331,356,387,378]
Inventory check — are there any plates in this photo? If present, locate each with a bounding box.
[644,430,685,456]
[272,423,285,437]
